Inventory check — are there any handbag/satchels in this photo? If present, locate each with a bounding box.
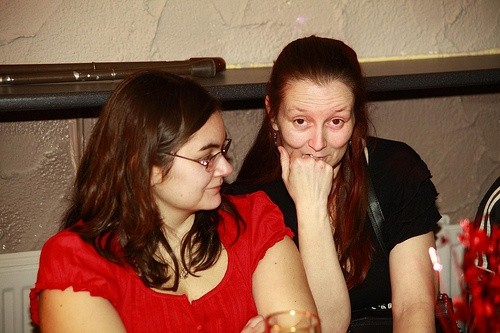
[434,292,460,333]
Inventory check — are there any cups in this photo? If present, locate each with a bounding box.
[264,310,319,333]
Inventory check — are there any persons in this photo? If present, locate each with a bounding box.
[29,68,321,333]
[224,35,443,333]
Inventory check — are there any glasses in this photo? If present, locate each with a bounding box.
[164,138,232,173]
